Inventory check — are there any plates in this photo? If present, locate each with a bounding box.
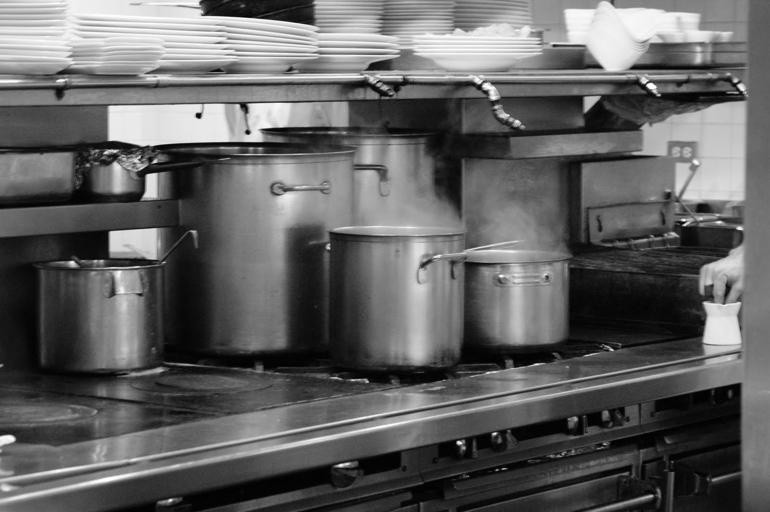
[1,0,73,76]
[412,33,544,75]
[71,34,164,74]
[62,10,237,74]
[456,1,540,30]
[306,0,386,36]
[215,15,321,74]
[387,0,458,67]
[289,32,403,71]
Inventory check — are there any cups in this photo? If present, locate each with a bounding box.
[702,300,743,349]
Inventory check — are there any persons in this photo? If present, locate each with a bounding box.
[694,242,744,307]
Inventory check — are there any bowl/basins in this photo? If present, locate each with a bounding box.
[565,6,734,42]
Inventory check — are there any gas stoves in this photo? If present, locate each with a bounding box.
[166,343,621,390]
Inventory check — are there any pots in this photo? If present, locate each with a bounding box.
[76,138,231,199]
[311,225,466,369]
[286,132,435,232]
[462,248,570,350]
[168,145,356,362]
[29,254,169,378]
